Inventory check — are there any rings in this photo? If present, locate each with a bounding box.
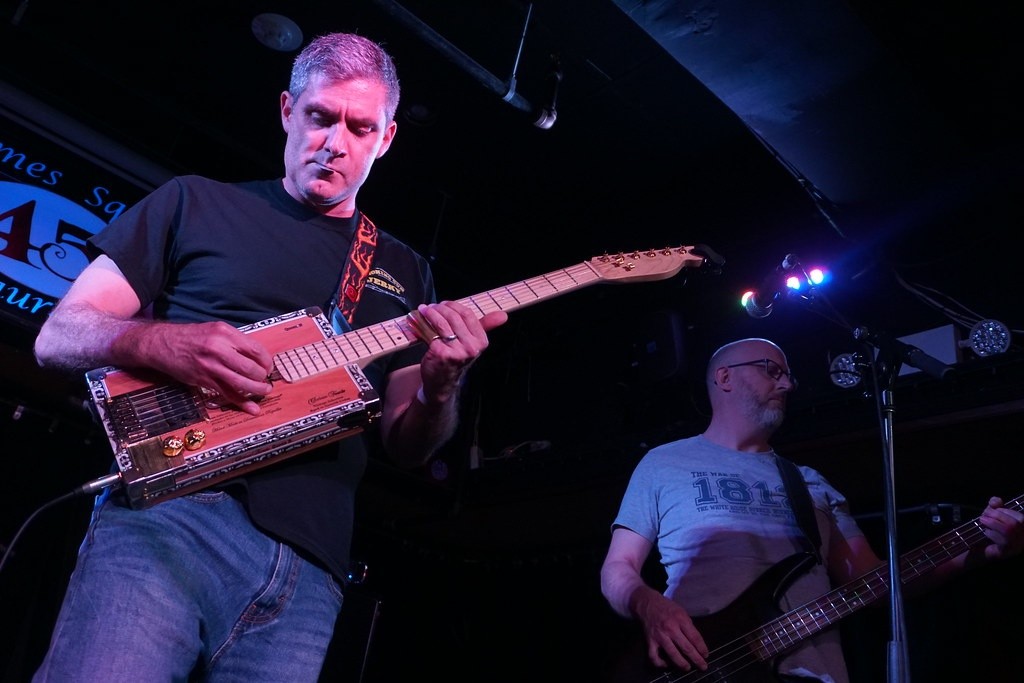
[428,334,440,342]
[443,334,457,340]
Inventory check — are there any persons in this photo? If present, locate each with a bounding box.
[600,338,1023,683]
[33,34,508,683]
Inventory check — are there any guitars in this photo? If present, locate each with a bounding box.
[83,242,727,512]
[640,492,1024,683]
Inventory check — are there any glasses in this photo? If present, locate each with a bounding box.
[713,359,797,386]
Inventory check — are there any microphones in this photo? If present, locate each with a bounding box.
[745,253,798,318]
